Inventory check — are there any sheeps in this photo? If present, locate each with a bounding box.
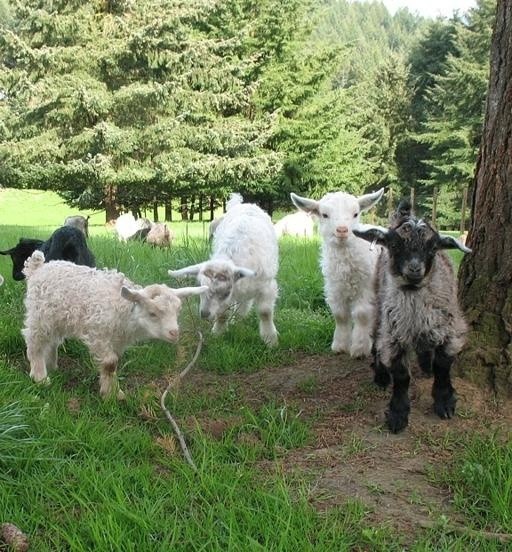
[146,221,169,246]
[274,210,315,239]
[0,224,97,282]
[289,186,390,361]
[165,191,281,350]
[63,215,90,239]
[114,212,149,244]
[350,207,475,436]
[18,247,210,406]
